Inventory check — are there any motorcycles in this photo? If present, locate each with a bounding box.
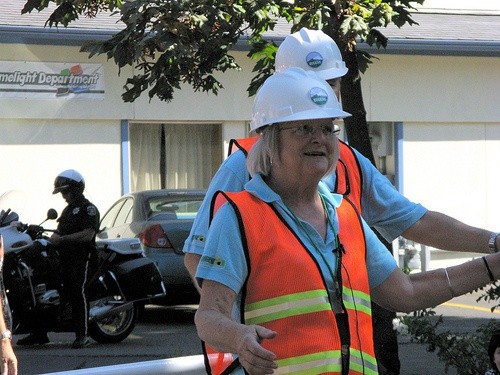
[0,209,166,346]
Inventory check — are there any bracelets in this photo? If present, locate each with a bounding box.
[0,330,12,340]
[444,269,455,297]
[489,233,499,254]
[61,237,64,240]
[482,255,494,281]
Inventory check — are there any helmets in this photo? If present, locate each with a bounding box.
[274,27,350,80]
[52,169,84,194]
[250,67,353,132]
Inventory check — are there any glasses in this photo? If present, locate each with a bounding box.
[279,123,340,140]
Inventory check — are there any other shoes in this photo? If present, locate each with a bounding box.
[70,336,91,348]
[15,332,50,345]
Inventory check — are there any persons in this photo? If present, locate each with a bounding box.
[485,334,500,375]
[0,234,17,375]
[183,27,500,375]
[16,169,100,346]
[194,67,500,375]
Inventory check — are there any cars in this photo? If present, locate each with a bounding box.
[93,188,211,316]
